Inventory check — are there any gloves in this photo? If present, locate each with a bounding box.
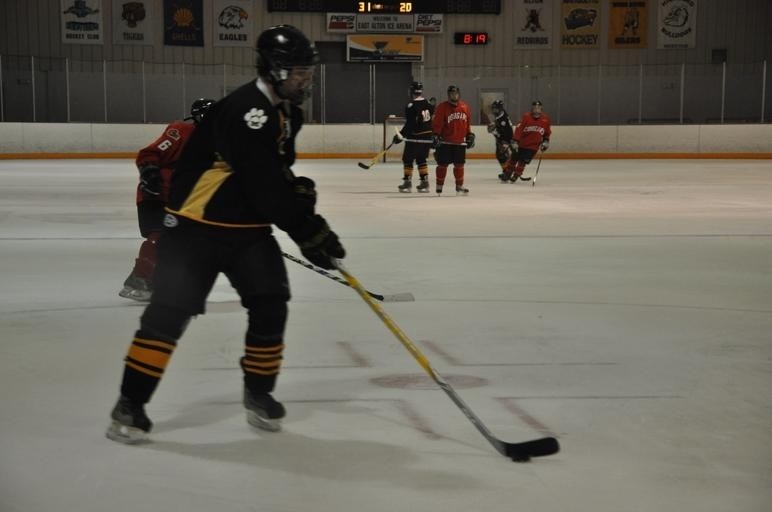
[293,176,316,213]
[467,133,475,148]
[140,164,163,201]
[392,134,402,144]
[293,215,345,269]
[432,136,444,149]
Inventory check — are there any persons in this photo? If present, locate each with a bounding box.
[392,82,435,193]
[103,23,347,446]
[118,97,217,302]
[429,97,437,106]
[498,100,552,184]
[431,84,476,197]
[488,100,514,179]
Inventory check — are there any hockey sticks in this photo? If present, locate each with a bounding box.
[533,149,545,183]
[358,142,394,169]
[491,131,531,180]
[281,251,414,303]
[324,251,559,462]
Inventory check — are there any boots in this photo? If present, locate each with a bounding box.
[399,176,412,188]
[416,174,428,188]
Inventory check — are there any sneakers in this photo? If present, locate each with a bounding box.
[456,186,469,193]
[111,397,149,429]
[125,271,154,291]
[498,172,517,181]
[244,387,283,418]
[436,184,442,193]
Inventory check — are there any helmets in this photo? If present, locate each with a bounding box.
[407,82,423,99]
[492,100,504,114]
[448,85,460,106]
[532,101,543,119]
[255,24,320,104]
[191,98,217,122]
